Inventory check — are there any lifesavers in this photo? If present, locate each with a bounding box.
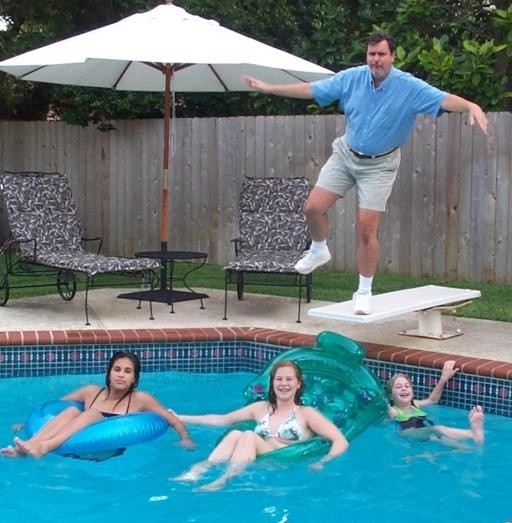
[24,397,170,456]
[222,327,390,465]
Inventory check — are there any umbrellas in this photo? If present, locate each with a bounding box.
[0,1,337,291]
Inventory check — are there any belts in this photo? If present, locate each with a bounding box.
[350,146,399,160]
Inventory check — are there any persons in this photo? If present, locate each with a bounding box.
[167,360,349,490]
[387,360,484,446]
[0,352,194,458]
[242,34,488,317]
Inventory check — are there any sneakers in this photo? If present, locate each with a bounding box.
[350,292,373,316]
[294,246,333,275]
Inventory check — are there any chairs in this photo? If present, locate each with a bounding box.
[221,172,312,325]
[1,169,160,327]
